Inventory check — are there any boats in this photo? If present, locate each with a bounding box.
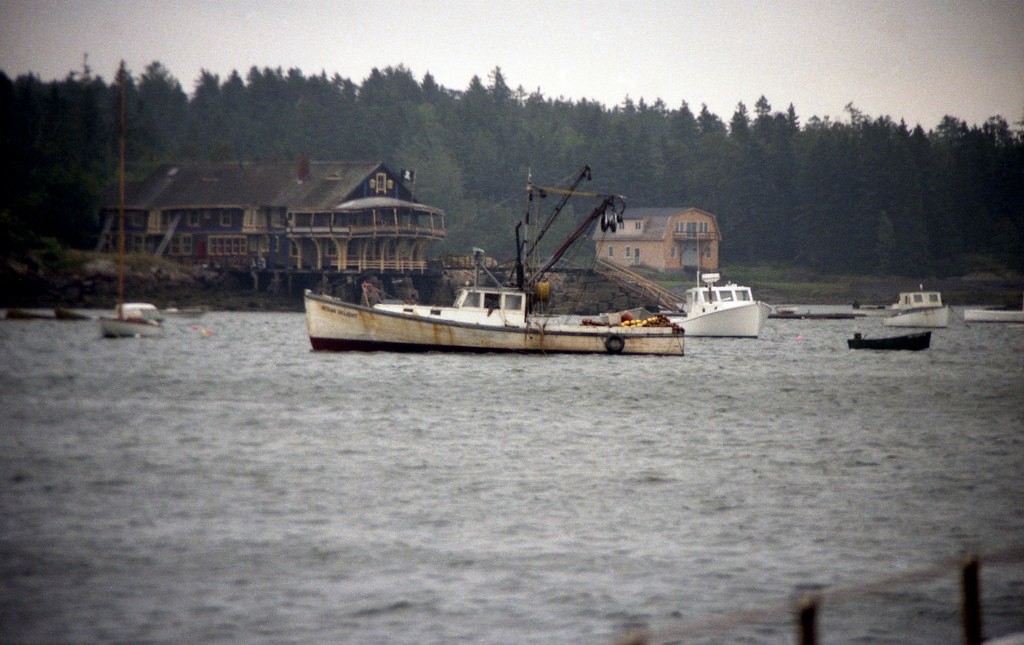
[882,284,949,329]
[962,305,1024,323]
[591,257,773,340]
[98,58,216,339]
[301,164,686,357]
[848,330,933,351]
